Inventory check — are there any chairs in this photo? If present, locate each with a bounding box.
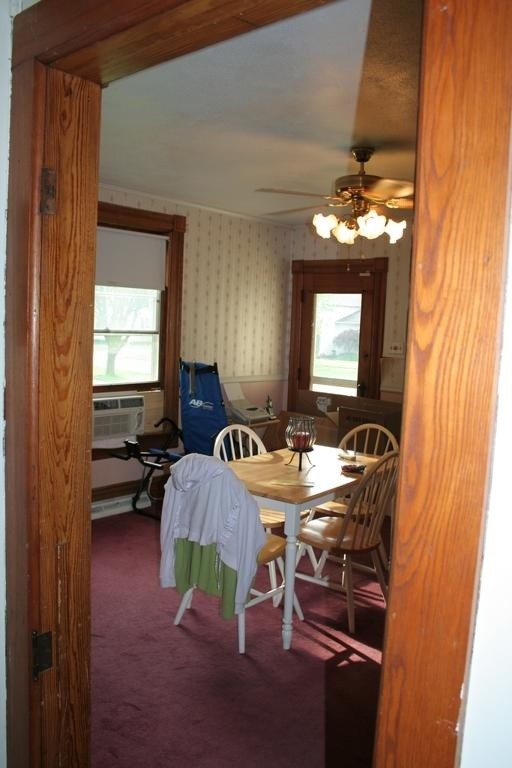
[306,423,399,588]
[174,454,305,655]
[275,449,400,634]
[213,425,321,597]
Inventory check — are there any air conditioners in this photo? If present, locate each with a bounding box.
[92,395,146,441]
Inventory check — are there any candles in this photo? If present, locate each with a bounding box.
[293,432,309,448]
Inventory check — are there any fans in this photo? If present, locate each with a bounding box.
[255,146,414,217]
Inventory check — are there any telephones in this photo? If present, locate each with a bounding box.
[266,395,277,420]
[231,404,270,425]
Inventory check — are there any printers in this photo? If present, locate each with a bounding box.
[224,399,271,425]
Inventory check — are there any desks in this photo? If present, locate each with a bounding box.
[233,419,281,451]
[227,444,398,650]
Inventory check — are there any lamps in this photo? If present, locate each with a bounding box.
[313,160,407,271]
